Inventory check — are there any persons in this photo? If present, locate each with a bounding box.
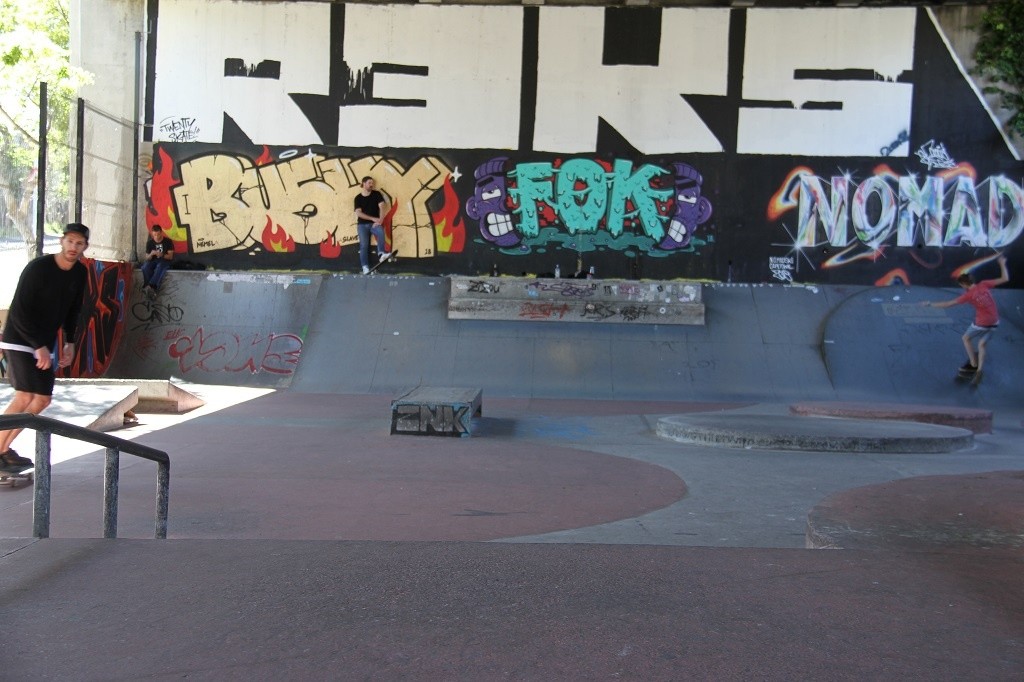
[2,221,94,469]
[139,225,174,299]
[921,258,1010,383]
[355,175,399,278]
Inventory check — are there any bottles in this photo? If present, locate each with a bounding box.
[587,266,595,280]
[554,264,560,280]
[493,264,498,277]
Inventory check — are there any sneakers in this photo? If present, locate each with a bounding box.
[959,364,978,372]
[362,265,370,274]
[378,252,391,263]
[0,448,34,472]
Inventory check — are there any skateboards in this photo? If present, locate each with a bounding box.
[957,351,979,380]
[0,462,37,487]
[366,249,398,275]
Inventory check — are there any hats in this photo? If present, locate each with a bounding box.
[63,223,90,239]
[360,176,373,188]
